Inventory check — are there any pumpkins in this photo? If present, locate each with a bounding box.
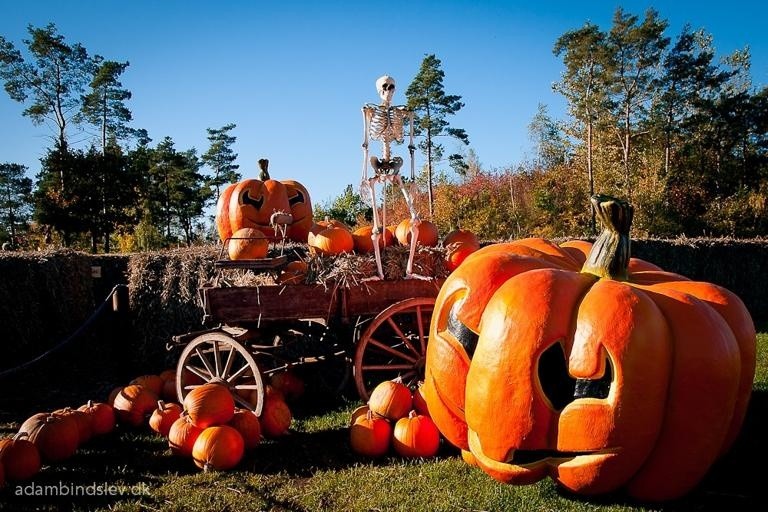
[348,378,440,457]
[0,365,303,489]
[424,192,756,501]
[215,158,481,285]
